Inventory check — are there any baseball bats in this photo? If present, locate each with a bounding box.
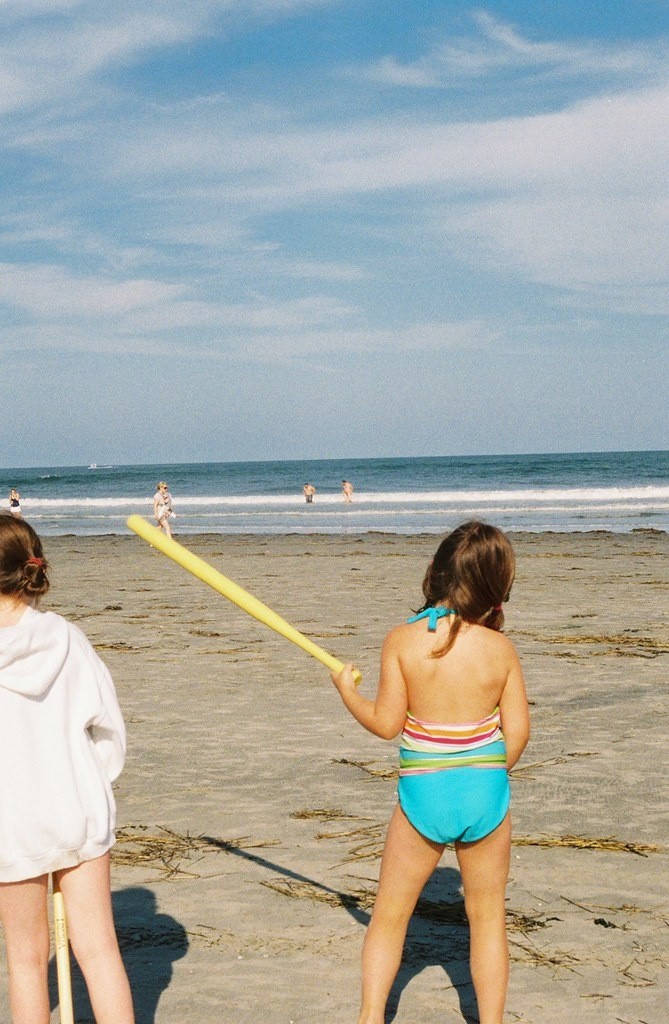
[125,514,363,687]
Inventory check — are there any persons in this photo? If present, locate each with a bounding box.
[9,489,21,516]
[0,512,135,1024]
[303,483,315,502]
[330,520,530,1024]
[341,480,353,502]
[150,482,173,548]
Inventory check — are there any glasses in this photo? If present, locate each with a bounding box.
[164,487,168,489]
